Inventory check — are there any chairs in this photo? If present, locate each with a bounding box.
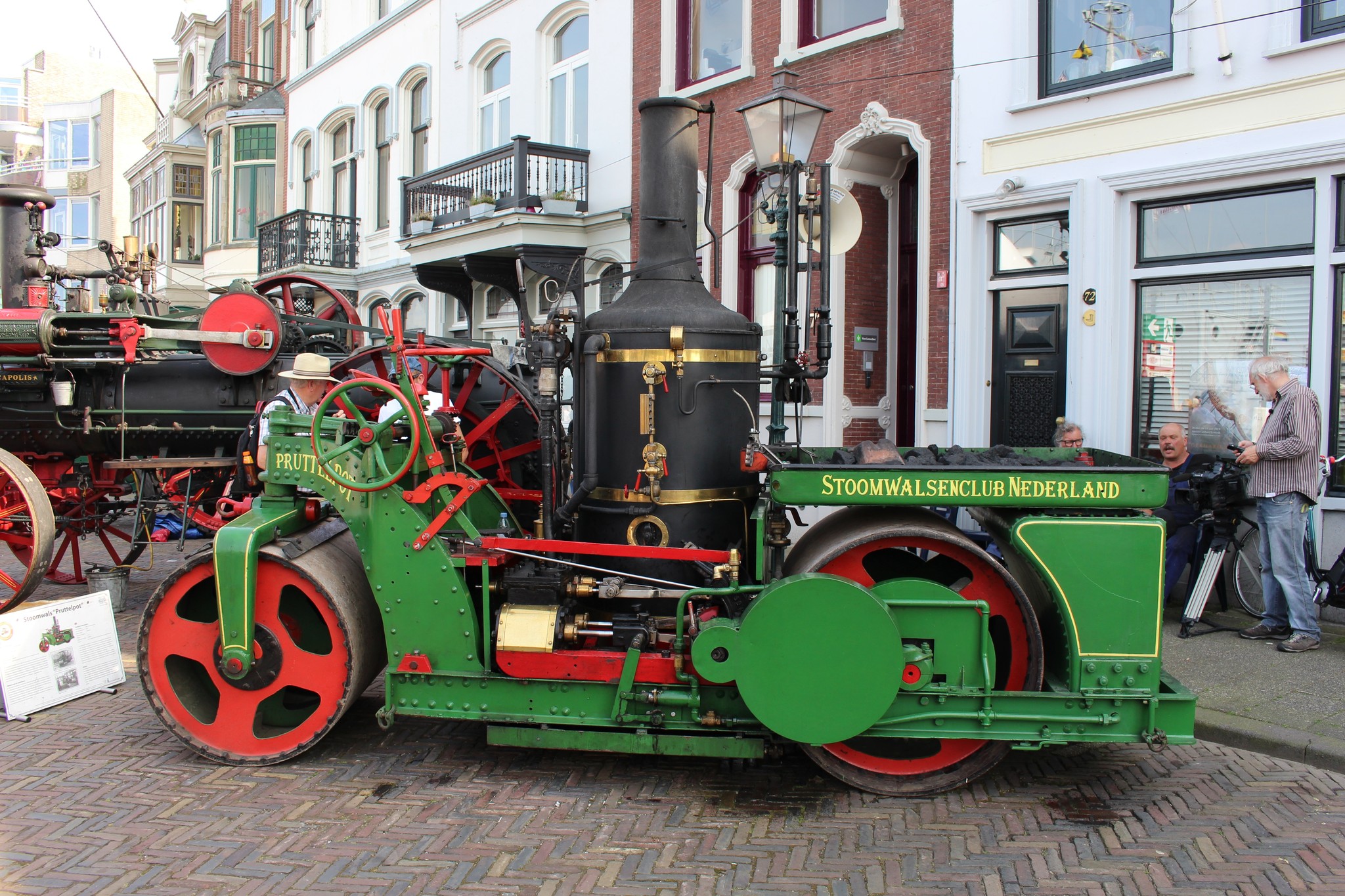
[1185,453,1228,623]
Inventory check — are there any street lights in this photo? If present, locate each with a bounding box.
[727,60,836,574]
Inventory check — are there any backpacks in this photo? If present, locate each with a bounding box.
[236,396,296,496]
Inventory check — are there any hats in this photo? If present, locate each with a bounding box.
[277,353,343,383]
[387,356,422,379]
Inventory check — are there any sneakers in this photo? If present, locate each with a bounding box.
[1276,633,1320,653]
[1237,622,1290,639]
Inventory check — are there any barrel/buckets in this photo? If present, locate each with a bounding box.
[51,368,77,406]
[83,561,131,613]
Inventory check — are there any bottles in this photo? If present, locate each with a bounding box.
[497,513,510,538]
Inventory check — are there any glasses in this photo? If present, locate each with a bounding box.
[1061,438,1083,446]
[390,373,420,385]
[1250,375,1257,390]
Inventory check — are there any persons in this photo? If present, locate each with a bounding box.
[1051,421,1086,450]
[253,351,341,495]
[378,353,469,463]
[1139,422,1210,612]
[1231,356,1323,655]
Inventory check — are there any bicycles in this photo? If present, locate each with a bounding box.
[1230,455,1345,624]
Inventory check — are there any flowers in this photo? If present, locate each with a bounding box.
[409,209,435,223]
[540,187,578,201]
[465,193,496,206]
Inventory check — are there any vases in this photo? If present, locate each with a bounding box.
[469,203,496,221]
[411,220,434,236]
[541,199,577,215]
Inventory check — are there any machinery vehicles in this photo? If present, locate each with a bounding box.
[0,182,572,617]
[138,90,1210,807]
